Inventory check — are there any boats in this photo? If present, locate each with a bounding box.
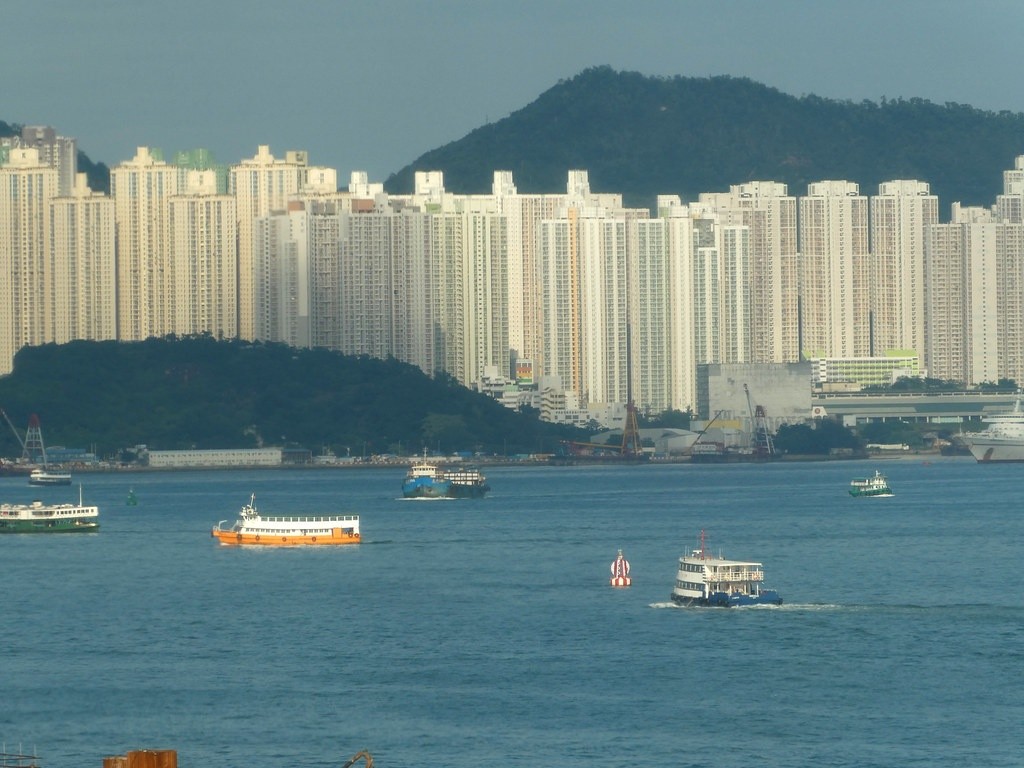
[400,445,491,499]
[671,530,784,607]
[946,394,1024,464]
[29,469,74,486]
[0,482,103,533]
[848,469,892,497]
[211,493,362,545]
